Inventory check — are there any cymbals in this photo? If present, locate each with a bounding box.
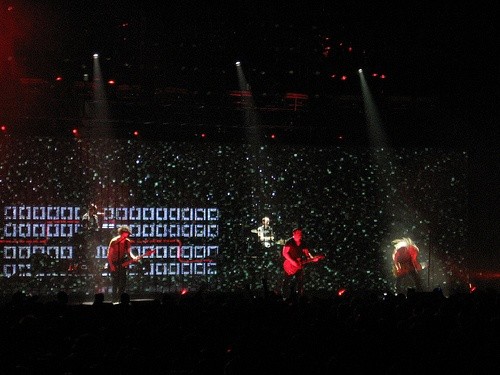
[252,230,269,234]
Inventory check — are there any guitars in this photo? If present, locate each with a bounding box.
[391,261,415,278]
[283,254,325,275]
[108,249,156,278]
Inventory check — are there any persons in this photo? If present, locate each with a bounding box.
[0,227,500,375]
[83,204,100,263]
[258,216,282,264]
